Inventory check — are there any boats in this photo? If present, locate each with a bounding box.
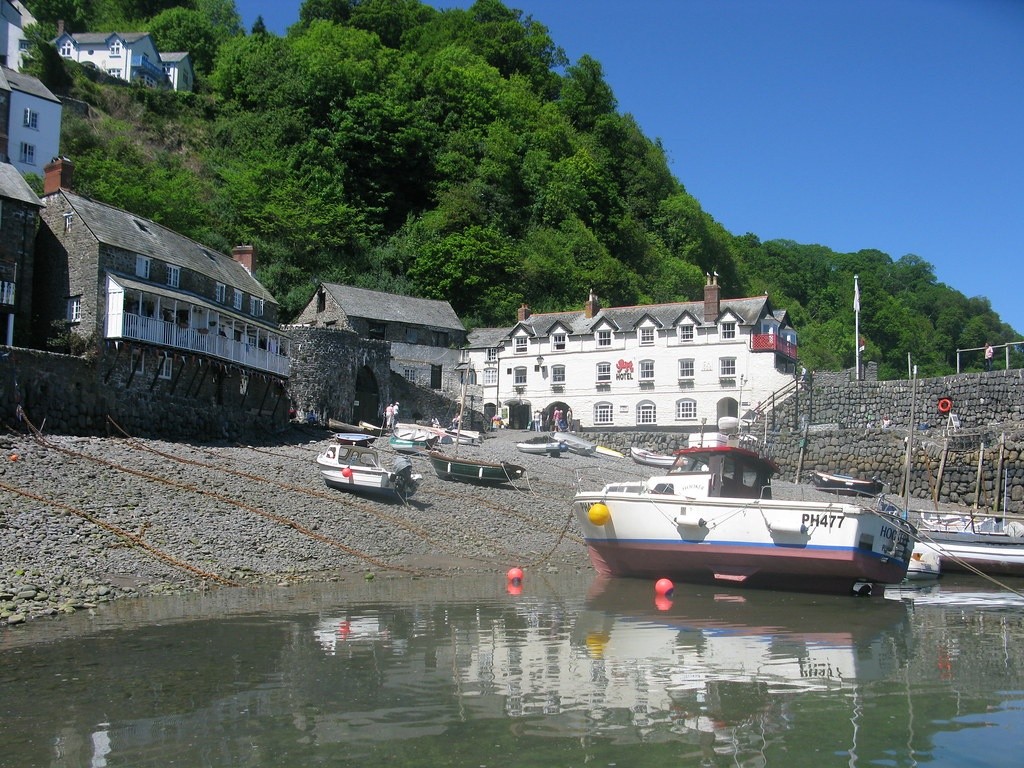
[810,469,886,499]
[328,418,366,435]
[516,435,569,455]
[550,430,600,457]
[390,421,485,455]
[897,510,1024,581]
[334,432,378,448]
[572,443,918,597]
[317,443,424,501]
[358,420,386,435]
[629,445,690,468]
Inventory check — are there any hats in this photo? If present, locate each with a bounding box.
[390,404,393,407]
[396,402,399,405]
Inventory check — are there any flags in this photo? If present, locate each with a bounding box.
[852,285,861,312]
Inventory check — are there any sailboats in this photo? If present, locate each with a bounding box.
[427,357,527,484]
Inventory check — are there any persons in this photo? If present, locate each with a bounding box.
[386,402,464,430]
[985,343,993,371]
[800,365,807,389]
[307,410,318,428]
[533,406,575,433]
[289,405,301,423]
[753,401,762,422]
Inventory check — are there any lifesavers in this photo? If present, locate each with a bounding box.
[938,399,951,412]
